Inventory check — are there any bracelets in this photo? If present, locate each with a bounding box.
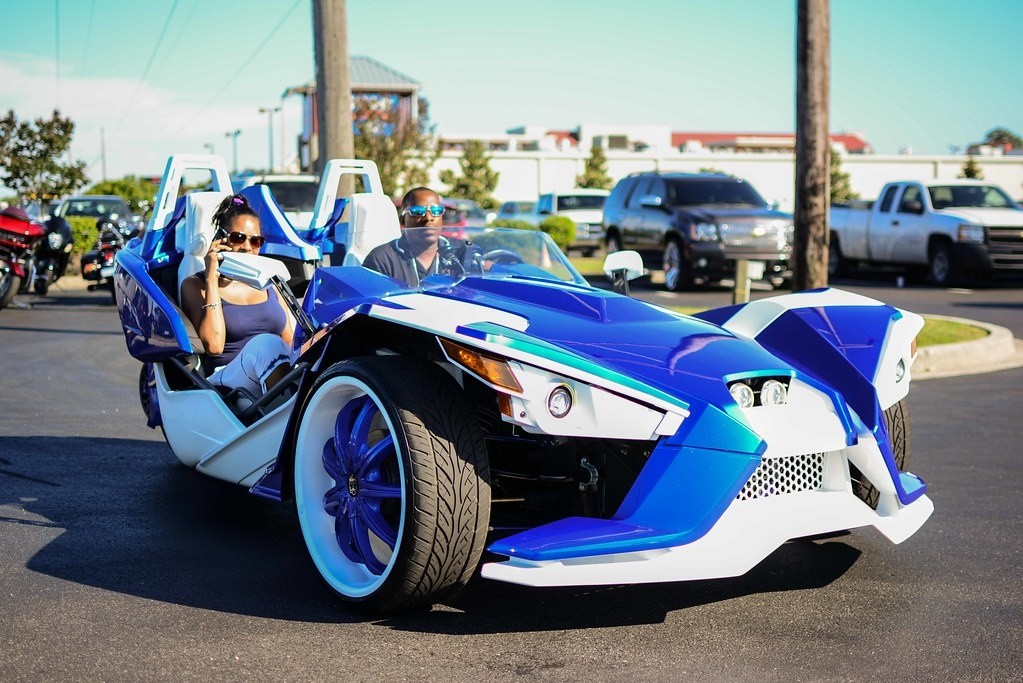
[202,302,221,308]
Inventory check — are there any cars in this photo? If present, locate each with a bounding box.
[24,196,130,229]
[602,172,794,292]
[243,175,320,228]
[389,197,488,241]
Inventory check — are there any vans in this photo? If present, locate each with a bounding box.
[496,187,611,257]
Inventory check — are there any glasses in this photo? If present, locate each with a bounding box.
[219,227,265,248]
[402,204,447,217]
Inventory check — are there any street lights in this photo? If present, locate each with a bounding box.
[225,130,241,171]
[259,107,281,170]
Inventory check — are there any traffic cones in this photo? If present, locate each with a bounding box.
[539,240,552,269]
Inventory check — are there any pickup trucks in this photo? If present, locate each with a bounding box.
[828,178,1023,289]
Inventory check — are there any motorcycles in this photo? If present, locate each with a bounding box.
[0,204,154,309]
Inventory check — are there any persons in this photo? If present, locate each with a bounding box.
[362,187,480,289]
[181,194,295,400]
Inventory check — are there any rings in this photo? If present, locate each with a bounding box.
[212,248,216,251]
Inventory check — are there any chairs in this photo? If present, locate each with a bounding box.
[342,192,403,266]
[178,192,231,310]
